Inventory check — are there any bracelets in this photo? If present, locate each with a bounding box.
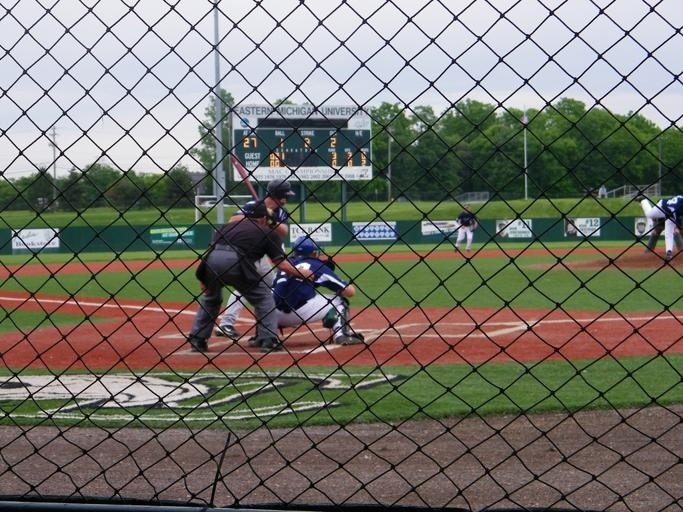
[269,220,276,226]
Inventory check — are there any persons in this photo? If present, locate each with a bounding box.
[453,204,477,251]
[248,235,364,348]
[636,195,683,260]
[215,178,290,337]
[644,199,682,253]
[597,185,607,198]
[188,202,313,352]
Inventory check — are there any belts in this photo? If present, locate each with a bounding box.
[214,244,239,252]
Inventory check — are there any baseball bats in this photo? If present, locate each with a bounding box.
[231,153,258,200]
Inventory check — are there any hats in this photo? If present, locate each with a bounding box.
[243,200,273,220]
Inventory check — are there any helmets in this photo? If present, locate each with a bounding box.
[265,180,295,196]
[293,236,320,254]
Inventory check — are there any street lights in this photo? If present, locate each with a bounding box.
[516,113,529,200]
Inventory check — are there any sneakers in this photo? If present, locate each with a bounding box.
[260,338,281,352]
[216,325,239,337]
[248,337,256,347]
[666,251,670,260]
[334,334,361,345]
[190,337,207,352]
[634,196,644,201]
